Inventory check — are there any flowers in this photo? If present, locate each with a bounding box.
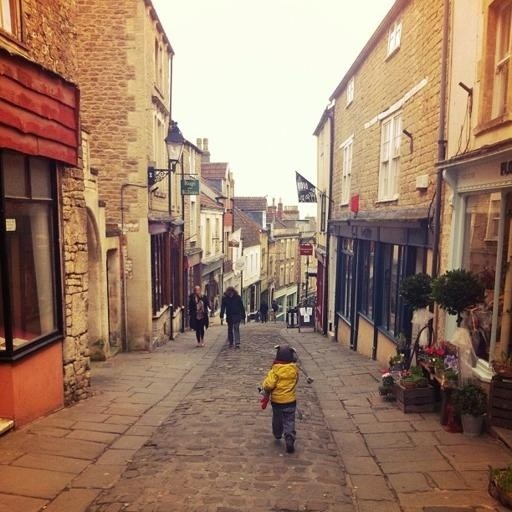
[417,340,460,391]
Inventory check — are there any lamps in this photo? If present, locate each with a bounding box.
[148,123,186,190]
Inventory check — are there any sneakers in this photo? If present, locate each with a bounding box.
[284,435,295,453]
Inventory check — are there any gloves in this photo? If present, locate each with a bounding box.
[257,389,268,409]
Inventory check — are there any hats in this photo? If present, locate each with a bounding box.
[275,344,297,361]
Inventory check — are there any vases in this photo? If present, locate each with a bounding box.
[439,388,451,425]
[444,390,460,433]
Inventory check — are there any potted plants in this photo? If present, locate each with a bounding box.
[460,384,486,436]
[490,465,512,504]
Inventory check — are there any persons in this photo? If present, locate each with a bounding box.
[205,274,219,317]
[220,286,246,348]
[272,299,279,324]
[260,300,268,324]
[260,343,300,454]
[188,285,212,347]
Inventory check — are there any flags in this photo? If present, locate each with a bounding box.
[294,171,318,204]
